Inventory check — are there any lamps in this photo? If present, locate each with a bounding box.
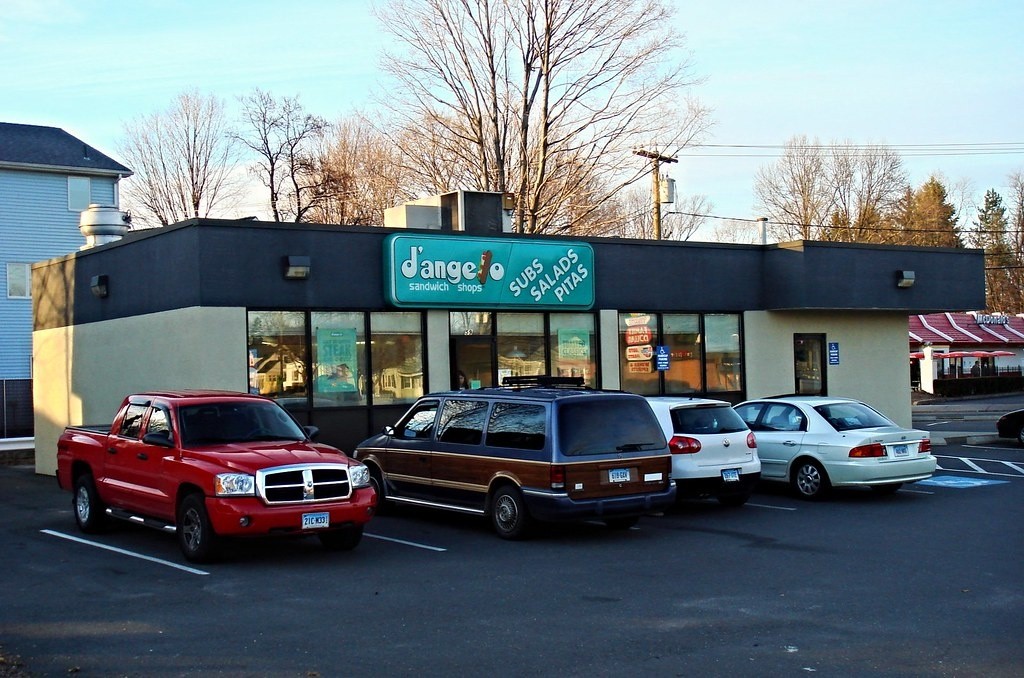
[285,256,311,279]
[898,271,916,287]
[91,276,108,297]
[657,174,676,204]
[507,345,525,358]
[661,211,768,222]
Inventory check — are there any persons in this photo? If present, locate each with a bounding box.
[457,371,469,391]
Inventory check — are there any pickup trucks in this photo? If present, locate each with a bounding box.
[56,389,378,565]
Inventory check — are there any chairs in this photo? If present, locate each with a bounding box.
[823,408,838,426]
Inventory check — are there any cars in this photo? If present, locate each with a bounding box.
[562,396,763,518]
[353,375,676,539]
[689,395,938,503]
[996,407,1024,443]
